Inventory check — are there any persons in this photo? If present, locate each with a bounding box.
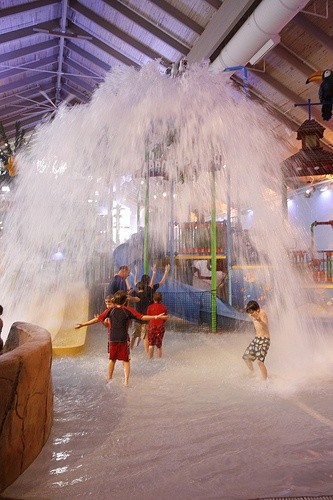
[241,300,271,381]
[125,263,157,353]
[134,264,171,346]
[75,290,169,386]
[104,266,130,307]
[146,292,167,360]
[94,294,114,353]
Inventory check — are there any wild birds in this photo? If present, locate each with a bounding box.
[305,69,333,121]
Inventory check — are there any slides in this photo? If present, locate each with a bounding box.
[113,241,333,339]
[52,278,88,357]
